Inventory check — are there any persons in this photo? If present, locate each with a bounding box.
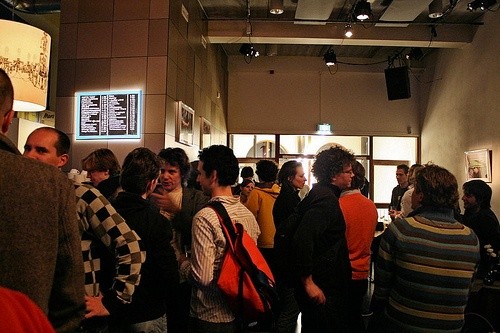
[0,68,87,333]
[81,147,179,333]
[23,127,146,333]
[0,287,56,333]
[179,146,306,333]
[286,148,378,333]
[370,164,500,333]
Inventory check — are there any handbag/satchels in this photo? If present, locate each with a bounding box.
[205,200,279,320]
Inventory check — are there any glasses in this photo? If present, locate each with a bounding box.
[336,168,353,174]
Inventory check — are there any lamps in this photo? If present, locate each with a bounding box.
[239,43,260,58]
[355,0,371,21]
[268,0,284,15]
[0,19,52,113]
[324,48,337,67]
[429,0,444,19]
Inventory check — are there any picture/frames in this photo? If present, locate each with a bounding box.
[199,116,212,150]
[464,148,493,184]
[177,100,196,147]
